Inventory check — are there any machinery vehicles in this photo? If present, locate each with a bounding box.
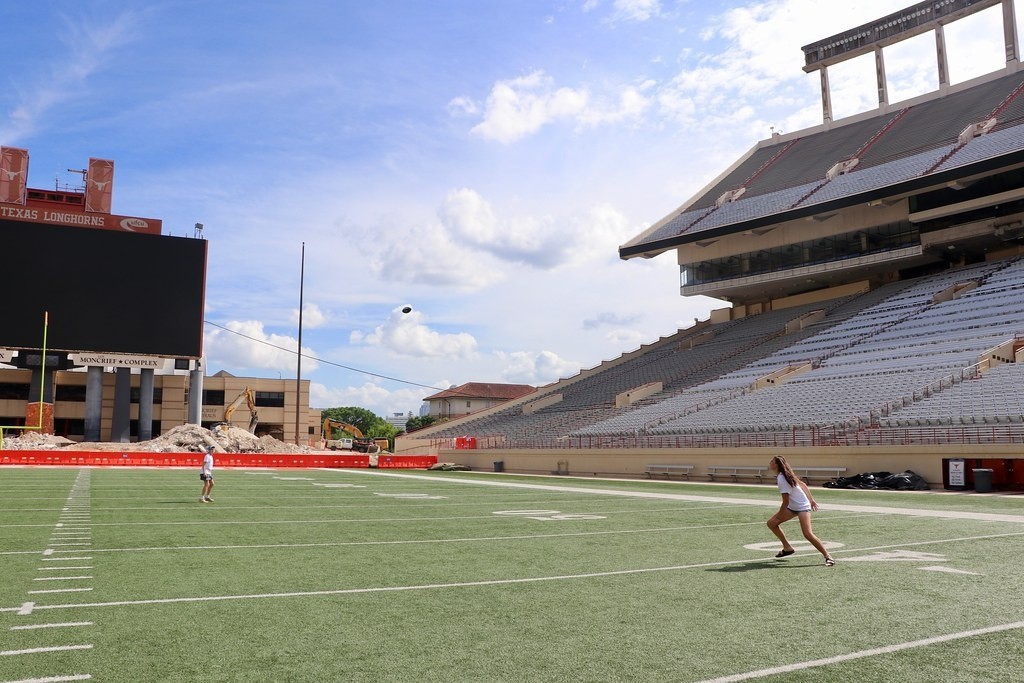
[210,386,259,432]
[324,418,383,453]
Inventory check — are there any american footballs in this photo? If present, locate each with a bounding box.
[402,306,412,313]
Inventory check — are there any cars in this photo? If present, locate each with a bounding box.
[338,438,352,448]
[449,464,471,471]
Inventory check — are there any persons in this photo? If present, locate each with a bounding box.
[199,446,215,502]
[766,455,835,566]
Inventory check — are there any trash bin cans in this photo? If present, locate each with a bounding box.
[1008,458,1024,491]
[557,462,568,475]
[972,468,994,493]
[943,458,977,491]
[981,458,1009,489]
[494,462,503,472]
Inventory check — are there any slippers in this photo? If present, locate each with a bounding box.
[825,558,835,566]
[775,549,795,557]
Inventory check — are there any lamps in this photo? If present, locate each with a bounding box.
[196,223,203,230]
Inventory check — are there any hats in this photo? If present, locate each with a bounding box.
[207,445,215,450]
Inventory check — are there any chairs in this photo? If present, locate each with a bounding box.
[429,257,1024,445]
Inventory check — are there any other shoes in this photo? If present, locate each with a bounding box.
[199,498,208,504]
[205,497,214,502]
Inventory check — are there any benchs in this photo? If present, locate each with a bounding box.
[773,467,847,486]
[707,466,769,484]
[645,464,695,481]
